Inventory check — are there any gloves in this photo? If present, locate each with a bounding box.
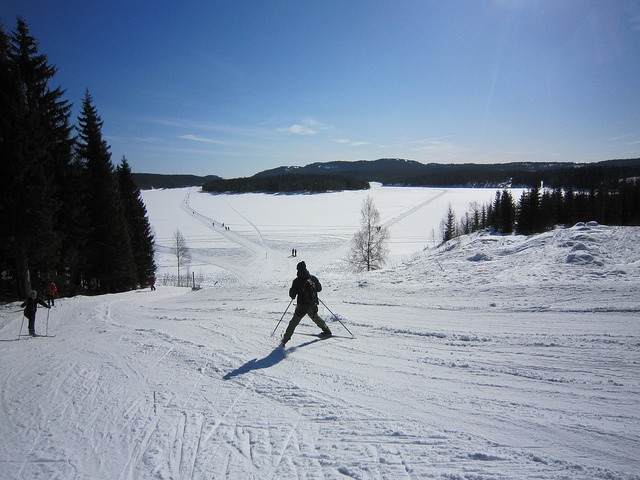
[25,301,30,305]
[47,306,51,309]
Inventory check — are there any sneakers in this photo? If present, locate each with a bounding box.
[32,332,37,336]
[29,331,32,335]
[320,331,331,336]
[282,337,289,343]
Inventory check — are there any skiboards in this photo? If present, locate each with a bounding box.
[17,333,54,338]
[280,333,357,349]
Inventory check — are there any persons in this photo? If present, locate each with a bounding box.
[44,279,58,307]
[21,289,51,337]
[279,262,331,348]
[147,271,157,291]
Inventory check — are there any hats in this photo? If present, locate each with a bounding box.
[30,291,36,296]
[297,261,306,271]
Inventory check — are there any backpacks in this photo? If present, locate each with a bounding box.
[302,280,317,306]
